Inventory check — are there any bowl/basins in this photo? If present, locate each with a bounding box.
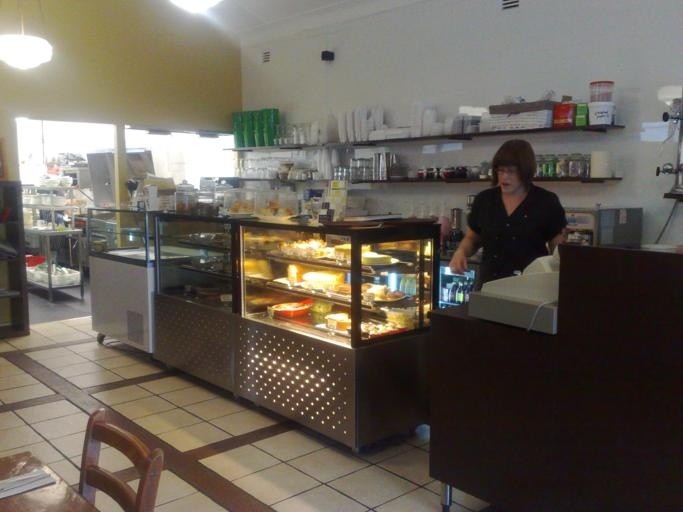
[272,301,310,317]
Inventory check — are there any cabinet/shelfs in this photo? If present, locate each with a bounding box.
[22,186,85,303]
[221,122,627,186]
[152,208,442,457]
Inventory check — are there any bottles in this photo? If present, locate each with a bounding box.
[477,152,591,178]
[174,180,199,216]
[332,151,399,183]
[231,108,279,147]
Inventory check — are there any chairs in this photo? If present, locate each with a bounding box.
[78,409,165,512]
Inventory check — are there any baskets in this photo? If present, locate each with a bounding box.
[488,99,561,131]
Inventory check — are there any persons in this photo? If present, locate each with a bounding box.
[448,138,569,293]
